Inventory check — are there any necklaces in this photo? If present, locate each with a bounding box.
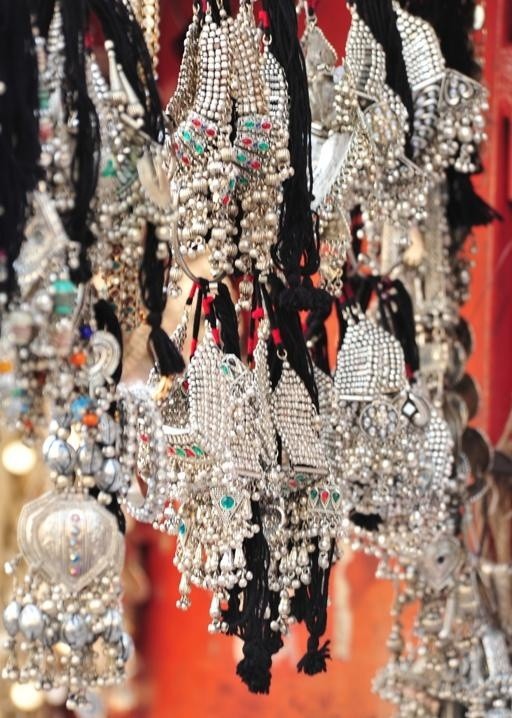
[0,0,512,718]
